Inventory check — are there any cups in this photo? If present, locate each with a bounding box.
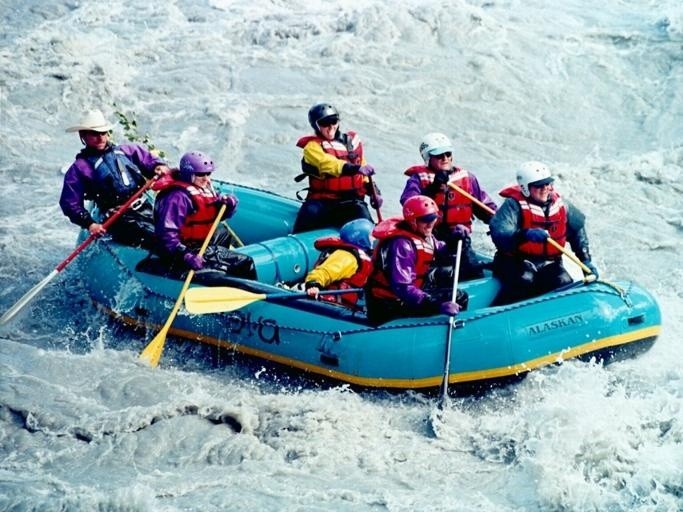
[315,118,339,128]
[193,171,212,178]
[432,151,452,159]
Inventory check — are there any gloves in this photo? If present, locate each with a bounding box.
[76,177,661,394]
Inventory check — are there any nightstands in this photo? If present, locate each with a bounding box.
[429,237,462,437]
[138,204,226,367]
[1,174,159,326]
[183,285,361,315]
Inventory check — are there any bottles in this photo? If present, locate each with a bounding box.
[64,109,121,133]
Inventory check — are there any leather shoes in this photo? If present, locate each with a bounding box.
[452,223,472,244]
[357,163,376,176]
[219,193,239,208]
[523,227,550,242]
[183,252,208,271]
[436,300,462,316]
[370,192,384,209]
[432,169,449,189]
[582,260,599,285]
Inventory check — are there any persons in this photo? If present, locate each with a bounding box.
[152,150,256,281]
[292,103,383,233]
[304,218,379,308]
[399,132,498,278]
[488,162,599,306]
[362,195,471,316]
[59,110,171,249]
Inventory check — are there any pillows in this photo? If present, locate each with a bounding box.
[515,160,554,198]
[339,217,376,252]
[179,150,216,183]
[307,102,341,131]
[418,132,454,167]
[402,194,442,232]
